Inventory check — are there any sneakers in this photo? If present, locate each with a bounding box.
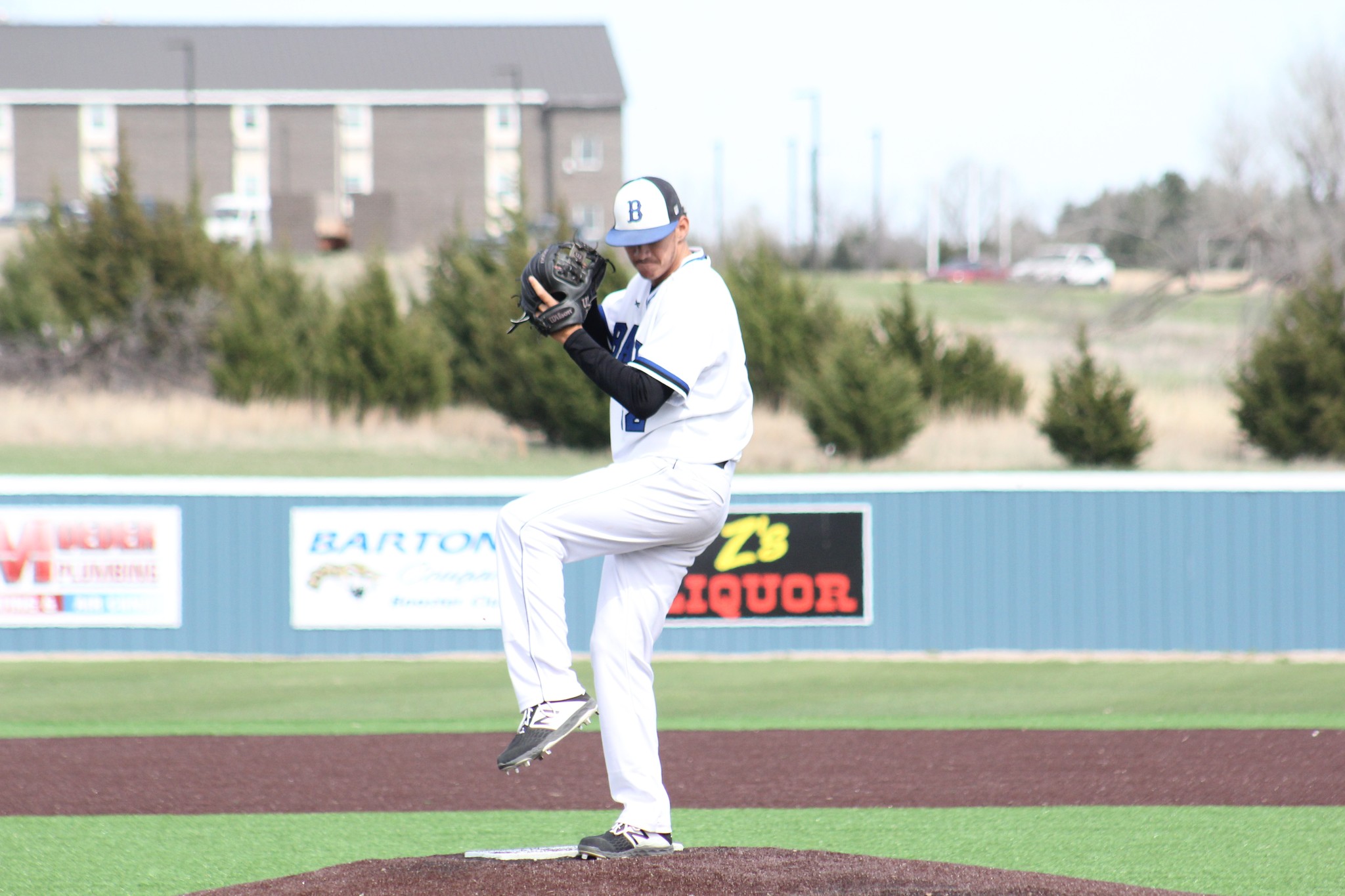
[578,823,673,860]
[497,692,599,775]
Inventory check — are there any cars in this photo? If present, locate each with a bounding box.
[1009,245,1118,287]
[927,256,1006,285]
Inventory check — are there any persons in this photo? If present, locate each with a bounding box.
[313,218,364,312]
[495,176,754,860]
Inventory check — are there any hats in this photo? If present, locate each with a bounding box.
[605,177,681,247]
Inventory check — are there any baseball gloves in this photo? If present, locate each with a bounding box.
[508,226,620,337]
